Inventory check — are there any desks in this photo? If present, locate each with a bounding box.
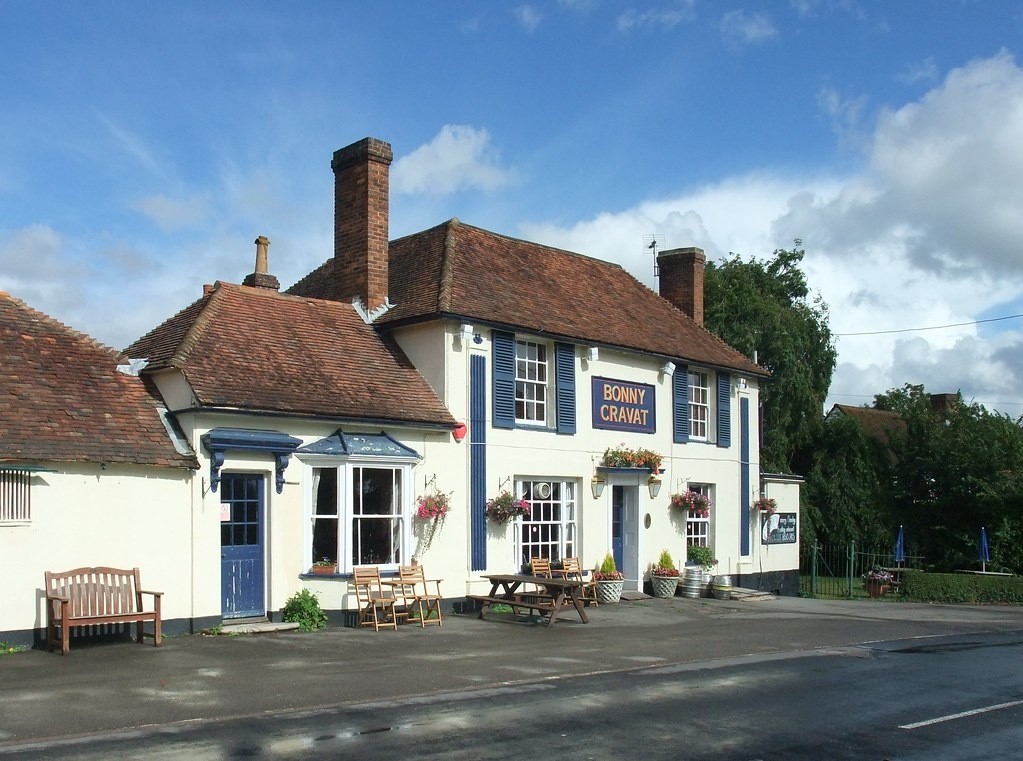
[477,575,589,628]
[550,569,579,604]
[860,568,925,593]
[381,580,421,624]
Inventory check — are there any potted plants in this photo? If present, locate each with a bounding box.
[411,555,419,571]
[594,551,624,603]
[312,559,337,575]
[649,549,680,598]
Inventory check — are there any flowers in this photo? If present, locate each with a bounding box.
[669,491,713,518]
[861,571,893,584]
[650,567,679,577]
[752,498,778,555]
[484,490,531,526]
[594,571,624,581]
[417,477,454,558]
[603,442,666,476]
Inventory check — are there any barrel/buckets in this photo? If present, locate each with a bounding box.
[701,574,713,598]
[681,568,702,598]
[711,575,732,599]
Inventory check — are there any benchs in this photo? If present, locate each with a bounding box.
[466,594,557,611]
[45,567,164,656]
[890,581,901,586]
[515,592,600,601]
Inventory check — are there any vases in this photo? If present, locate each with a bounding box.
[865,579,888,598]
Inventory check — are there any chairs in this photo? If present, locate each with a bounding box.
[561,558,599,607]
[352,567,398,631]
[530,558,569,607]
[399,565,443,628]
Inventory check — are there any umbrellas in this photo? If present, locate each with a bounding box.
[979,527,989,572]
[895,524,903,592]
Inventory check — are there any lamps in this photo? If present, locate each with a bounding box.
[731,377,746,392]
[453,323,473,339]
[582,347,599,362]
[648,477,662,499]
[660,362,676,376]
[591,475,606,499]
